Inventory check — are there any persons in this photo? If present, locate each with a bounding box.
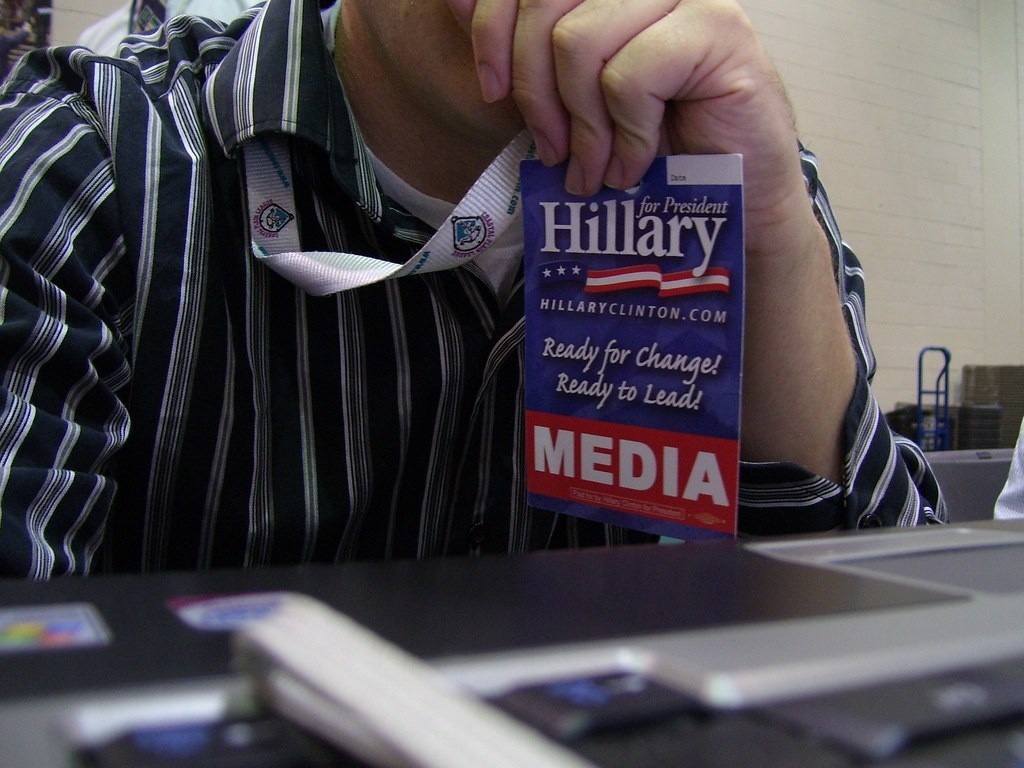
[0,0,950,583]
